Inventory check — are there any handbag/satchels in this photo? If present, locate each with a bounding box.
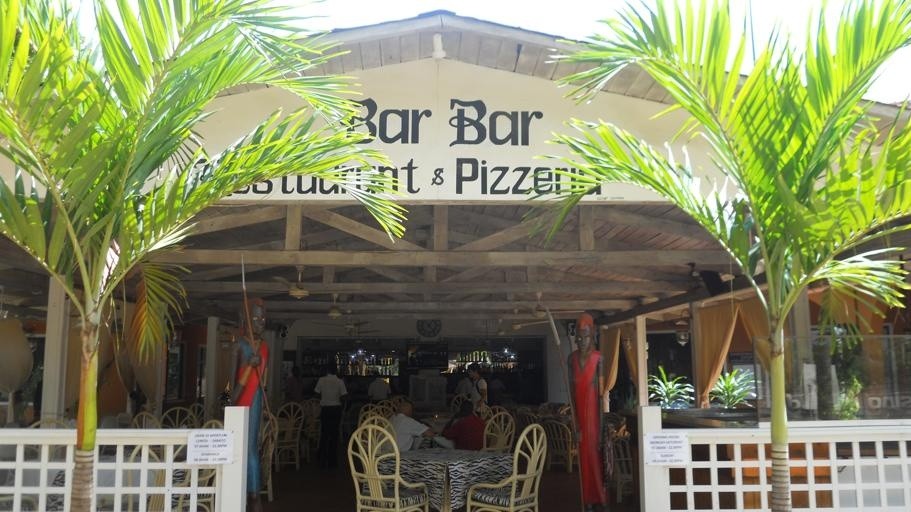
[476,378,494,407]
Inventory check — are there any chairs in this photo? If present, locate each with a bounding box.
[349,396,636,510]
[0,397,323,511]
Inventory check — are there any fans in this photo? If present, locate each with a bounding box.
[268,272,381,349]
[489,289,585,340]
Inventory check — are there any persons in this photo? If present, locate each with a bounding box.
[368,371,392,409]
[467,362,488,417]
[446,400,490,450]
[225,296,272,511]
[315,361,350,471]
[381,401,433,455]
[310,362,324,399]
[567,314,611,511]
[286,365,305,418]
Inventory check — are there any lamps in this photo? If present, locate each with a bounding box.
[671,308,693,347]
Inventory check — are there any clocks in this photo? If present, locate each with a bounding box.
[416,318,443,336]
